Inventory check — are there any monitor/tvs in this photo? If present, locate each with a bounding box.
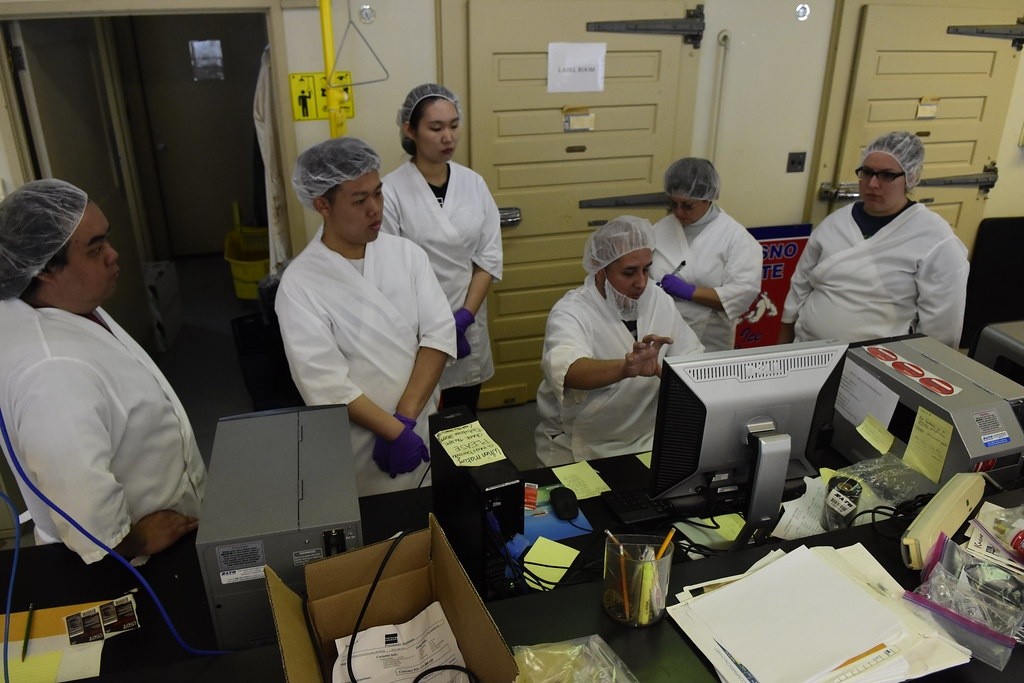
[647,339,849,552]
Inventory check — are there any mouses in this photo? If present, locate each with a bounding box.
[550,486,578,520]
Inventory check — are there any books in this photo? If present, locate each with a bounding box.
[465,453,523,493]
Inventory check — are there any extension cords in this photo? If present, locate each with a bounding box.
[899,473,985,570]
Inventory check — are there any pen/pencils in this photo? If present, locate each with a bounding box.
[619,544,630,619]
[661,261,687,287]
[656,528,676,560]
[21,603,33,662]
[605,529,633,559]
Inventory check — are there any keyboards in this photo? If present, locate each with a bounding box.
[602,485,671,525]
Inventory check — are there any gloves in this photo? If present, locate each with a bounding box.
[387,424,429,480]
[373,414,418,473]
[454,306,477,360]
[660,274,696,300]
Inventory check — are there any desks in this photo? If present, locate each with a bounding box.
[0,451,1024,683]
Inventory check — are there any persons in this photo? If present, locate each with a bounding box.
[534,216,706,468]
[648,157,763,354]
[379,83,503,419]
[274,137,457,498]
[774,130,970,351]
[0,178,207,565]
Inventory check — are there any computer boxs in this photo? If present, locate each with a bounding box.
[429,406,525,601]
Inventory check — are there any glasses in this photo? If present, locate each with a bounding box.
[855,166,906,184]
[663,200,701,211]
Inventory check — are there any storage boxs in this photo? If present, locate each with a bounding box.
[264,511,520,683]
